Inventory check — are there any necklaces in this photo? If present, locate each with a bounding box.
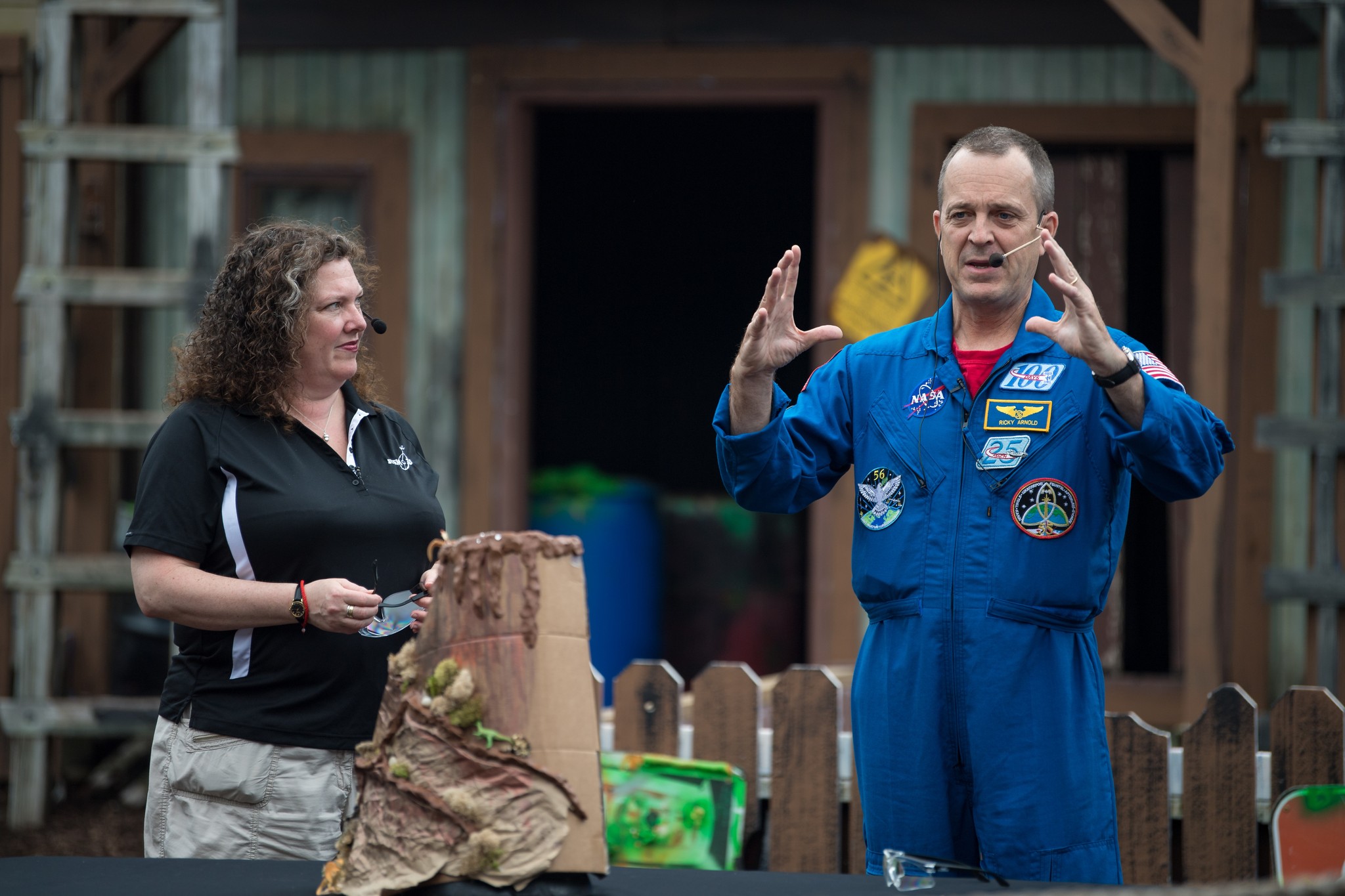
[282,398,333,441]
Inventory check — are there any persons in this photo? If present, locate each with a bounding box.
[712,127,1234,884]
[124,219,446,862]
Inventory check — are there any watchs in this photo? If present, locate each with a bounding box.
[289,583,307,624]
[1092,345,1142,388]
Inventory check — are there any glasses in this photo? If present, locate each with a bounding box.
[881,848,1012,890]
[358,558,431,638]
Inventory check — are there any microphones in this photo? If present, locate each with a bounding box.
[362,311,386,334]
[989,234,1042,268]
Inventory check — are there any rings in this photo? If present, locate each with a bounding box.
[346,605,354,618]
[1070,277,1078,285]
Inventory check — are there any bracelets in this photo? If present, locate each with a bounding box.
[300,580,309,634]
